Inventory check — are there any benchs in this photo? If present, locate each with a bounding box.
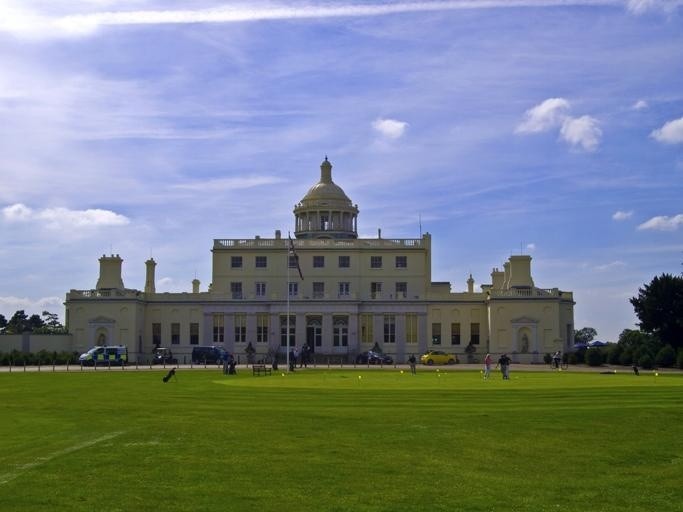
[252,365,271,376]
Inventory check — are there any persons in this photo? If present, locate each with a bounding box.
[407,352,416,375]
[496,355,506,378]
[271,355,279,372]
[503,354,512,380]
[229,355,237,375]
[288,345,313,372]
[220,351,229,375]
[552,350,563,373]
[482,354,492,378]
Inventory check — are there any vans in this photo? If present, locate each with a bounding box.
[190,345,234,366]
[78,344,128,367]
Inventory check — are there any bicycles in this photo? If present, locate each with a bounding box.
[548,357,568,370]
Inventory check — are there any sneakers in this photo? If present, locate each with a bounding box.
[485,372,489,377]
[412,371,416,374]
[502,375,509,380]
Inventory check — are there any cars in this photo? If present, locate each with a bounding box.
[151,347,173,364]
[419,350,457,366]
[355,350,393,366]
